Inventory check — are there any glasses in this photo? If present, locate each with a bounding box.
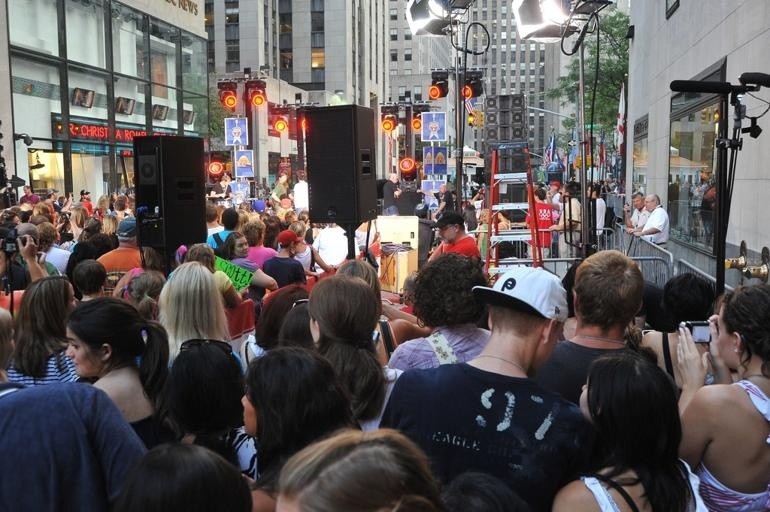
[439,225,454,233]
[179,338,233,356]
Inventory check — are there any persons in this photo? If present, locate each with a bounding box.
[156,261,246,445]
[620,188,649,234]
[633,193,668,252]
[387,252,493,371]
[442,468,535,511]
[8,278,86,387]
[414,162,621,235]
[274,429,440,512]
[302,273,407,430]
[106,441,251,512]
[183,245,312,344]
[1,173,429,263]
[240,348,361,512]
[0,234,164,322]
[429,208,481,262]
[64,298,168,449]
[676,284,770,512]
[641,271,720,389]
[376,265,606,512]
[532,248,680,474]
[162,343,257,483]
[1,367,148,512]
[554,351,702,512]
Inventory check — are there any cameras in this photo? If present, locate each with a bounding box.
[2,236,26,254]
[686,321,712,343]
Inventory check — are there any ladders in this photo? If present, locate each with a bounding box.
[486,142,543,272]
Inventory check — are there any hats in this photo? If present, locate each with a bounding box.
[252,199,266,215]
[275,229,305,246]
[430,211,466,232]
[80,189,91,195]
[469,264,569,326]
[44,189,59,198]
[18,209,33,223]
[115,216,136,238]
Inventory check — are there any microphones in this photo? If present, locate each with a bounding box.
[670,80,760,94]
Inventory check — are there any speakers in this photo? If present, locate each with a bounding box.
[305,104,377,224]
[133,135,207,246]
